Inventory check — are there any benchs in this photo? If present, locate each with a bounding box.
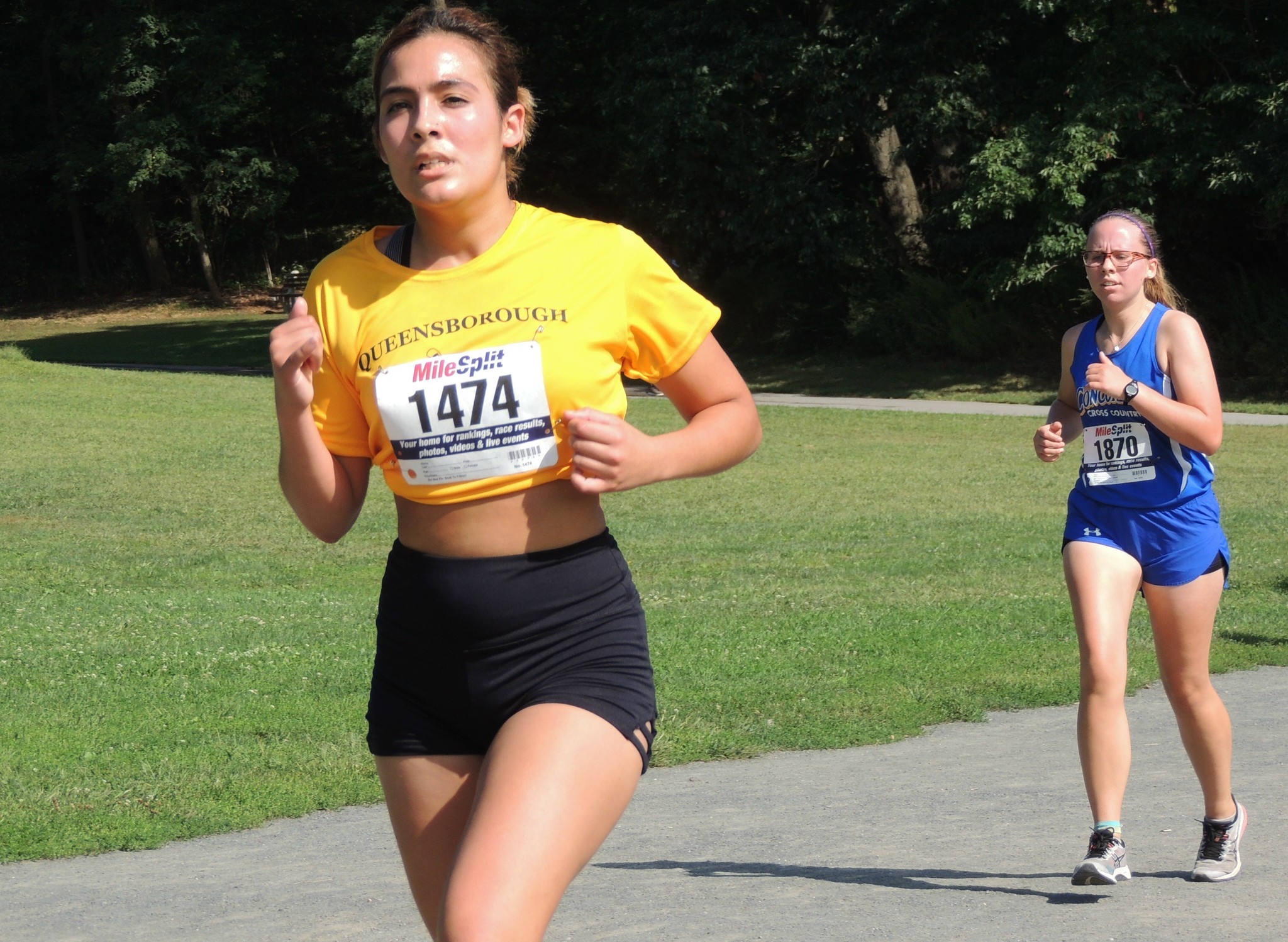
[268,272,311,314]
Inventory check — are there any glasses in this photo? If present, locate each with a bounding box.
[1081,250,1150,268]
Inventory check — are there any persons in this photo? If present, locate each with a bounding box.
[1033,209,1248,885]
[269,0,766,942]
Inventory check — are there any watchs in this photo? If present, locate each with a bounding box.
[1123,379,1138,405]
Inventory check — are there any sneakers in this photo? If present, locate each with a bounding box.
[1191,792,1248,882]
[1071,826,1131,885]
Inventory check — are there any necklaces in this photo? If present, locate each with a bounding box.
[1105,300,1149,351]
